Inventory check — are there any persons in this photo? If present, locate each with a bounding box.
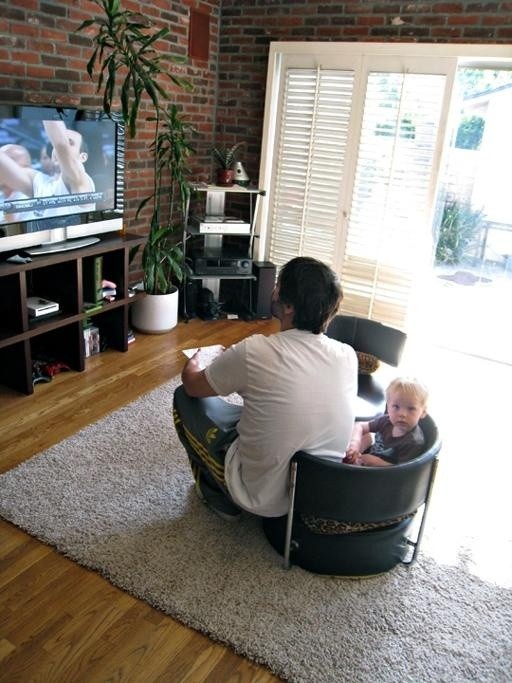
[0,144,31,222]
[40,142,54,177]
[0,120,95,219]
[342,377,428,467]
[174,256,358,523]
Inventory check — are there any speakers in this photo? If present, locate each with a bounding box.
[252,261,276,320]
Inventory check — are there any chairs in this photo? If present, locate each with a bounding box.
[324,314,408,367]
[261,400,442,575]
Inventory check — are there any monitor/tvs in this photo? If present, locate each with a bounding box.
[0,100,126,255]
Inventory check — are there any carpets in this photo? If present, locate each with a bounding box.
[1,350,512,683]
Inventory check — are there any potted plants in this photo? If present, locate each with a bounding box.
[69,0,206,335]
[213,139,247,187]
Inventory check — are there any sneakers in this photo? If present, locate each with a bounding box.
[194,479,244,522]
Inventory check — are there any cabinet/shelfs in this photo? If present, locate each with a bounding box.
[1,233,147,396]
[181,181,265,324]
